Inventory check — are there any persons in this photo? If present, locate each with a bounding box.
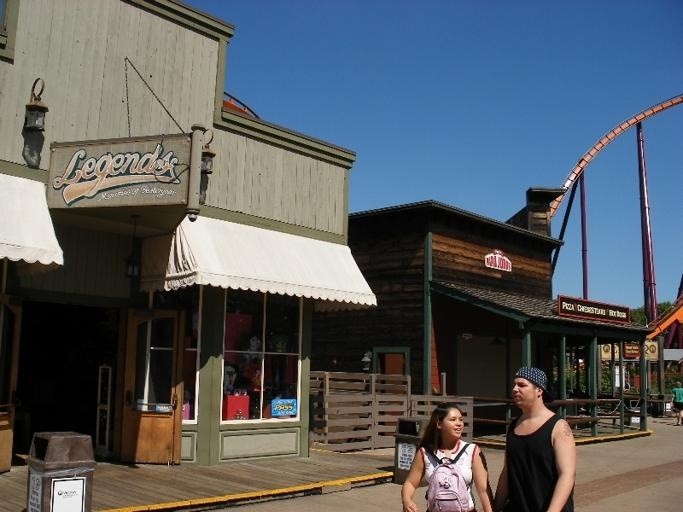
[492,366,578,511]
[669,381,682,425]
[399,403,493,512]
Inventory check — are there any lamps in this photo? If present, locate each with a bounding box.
[200,127,216,175]
[22,77,49,133]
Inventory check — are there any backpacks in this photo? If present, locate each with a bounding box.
[424,443,470,512]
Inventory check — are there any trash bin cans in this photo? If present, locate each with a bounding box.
[623,400,641,427]
[25,430,98,512]
[393,416,431,487]
[648,394,674,418]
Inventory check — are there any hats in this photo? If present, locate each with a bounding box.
[514,365,553,403]
